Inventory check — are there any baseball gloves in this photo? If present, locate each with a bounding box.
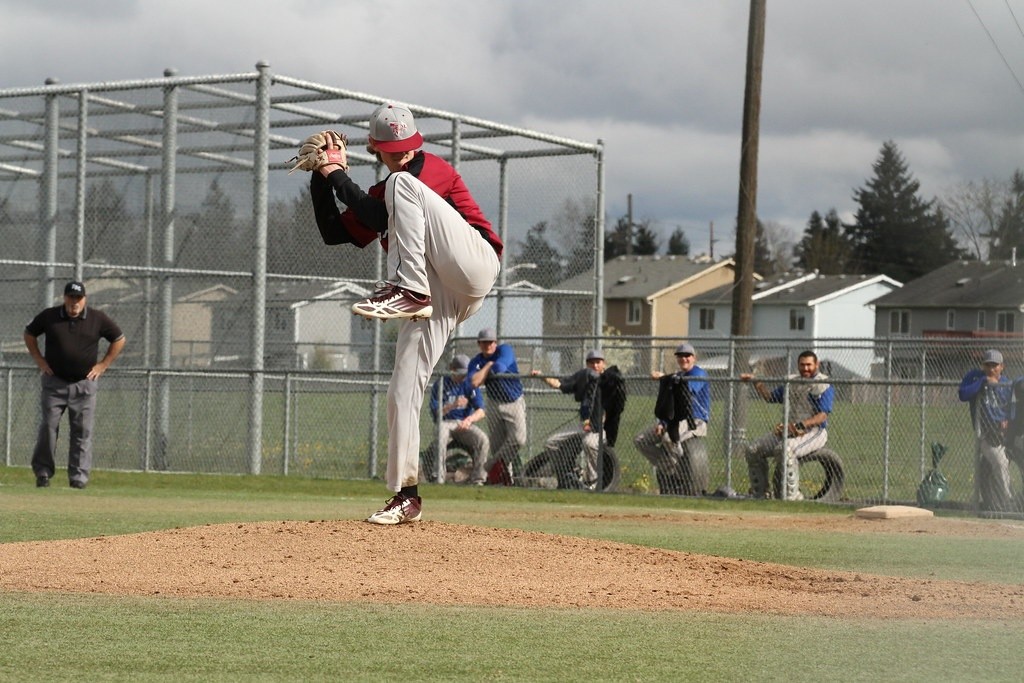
[283,129,350,177]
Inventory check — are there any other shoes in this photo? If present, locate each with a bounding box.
[474,479,483,487]
[70,480,84,489]
[36,475,50,487]
[585,480,597,491]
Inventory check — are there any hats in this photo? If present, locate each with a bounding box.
[477,328,497,341]
[64,282,86,298]
[982,349,1003,365]
[368,101,423,152]
[450,354,470,370]
[674,343,695,356]
[586,350,604,361]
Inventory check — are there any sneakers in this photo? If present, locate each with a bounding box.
[368,495,422,524]
[351,282,433,323]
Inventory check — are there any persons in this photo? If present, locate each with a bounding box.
[296,103,502,525]
[527,350,626,448]
[740,351,833,500]
[958,349,1024,511]
[650,344,711,496]
[422,354,489,486]
[23,282,125,486]
[468,328,526,487]
